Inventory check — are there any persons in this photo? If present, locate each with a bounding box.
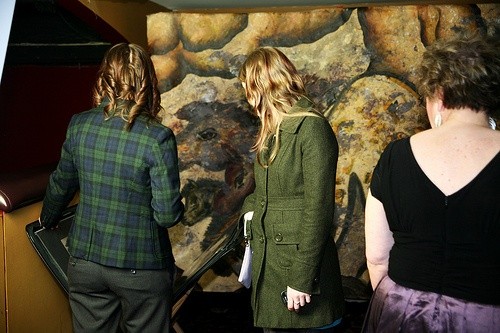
[235,47,347,333]
[39,43,186,332]
[363,40,500,333]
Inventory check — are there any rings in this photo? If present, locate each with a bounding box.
[294,301,300,305]
